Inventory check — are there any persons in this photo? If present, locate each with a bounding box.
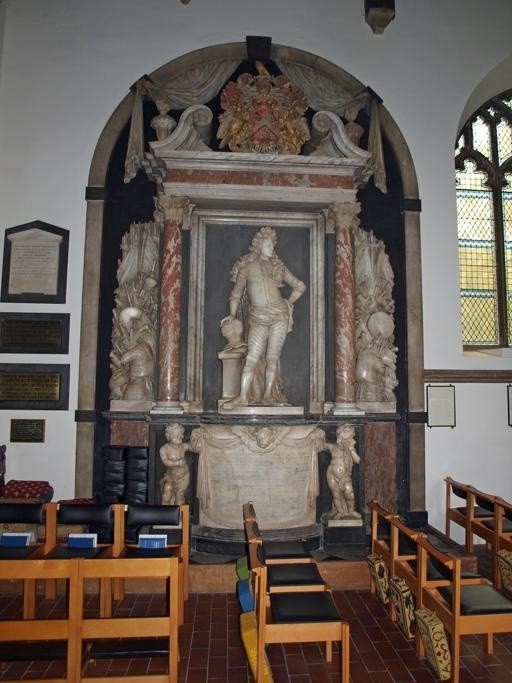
[220,226,308,410]
[160,423,202,508]
[315,422,361,520]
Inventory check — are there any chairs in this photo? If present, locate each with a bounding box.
[0,497,190,683]
[0,445,53,504]
[236,502,350,682]
[367,475,512,683]
